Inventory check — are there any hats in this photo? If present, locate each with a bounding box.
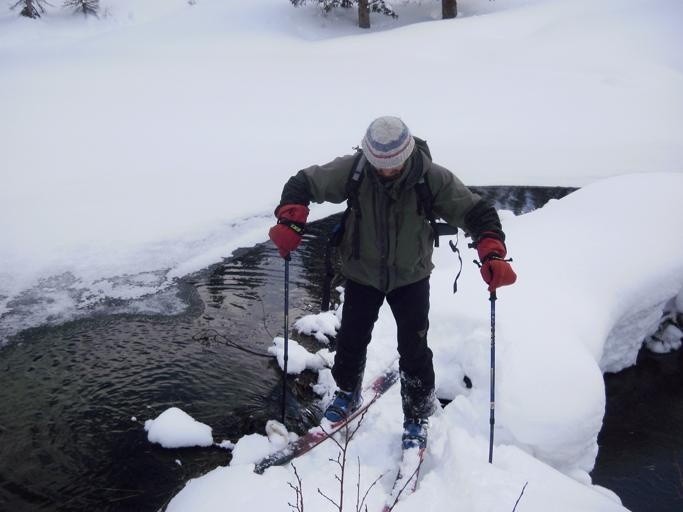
[361,116,416,170]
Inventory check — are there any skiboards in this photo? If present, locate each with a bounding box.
[254,353,472,512]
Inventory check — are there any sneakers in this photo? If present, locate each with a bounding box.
[325,389,364,422]
[402,414,429,449]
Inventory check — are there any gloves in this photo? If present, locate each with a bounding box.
[268,204,310,261]
[468,230,517,294]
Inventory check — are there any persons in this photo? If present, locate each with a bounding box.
[268,116,517,450]
